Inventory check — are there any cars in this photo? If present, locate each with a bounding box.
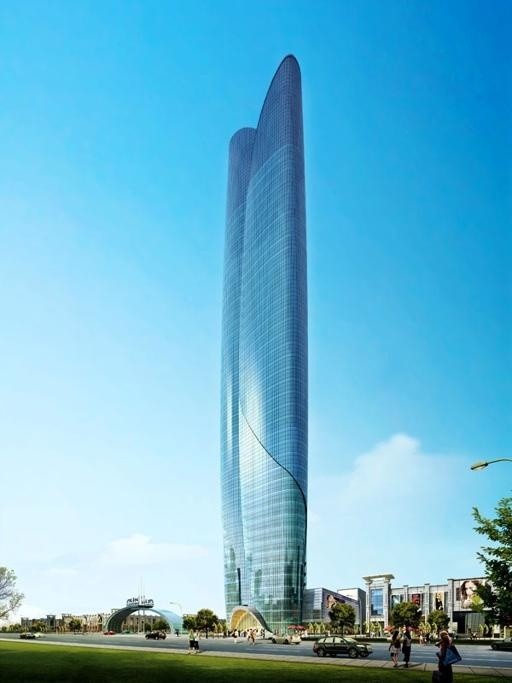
[491,637,512,651]
[104,630,115,635]
[145,629,166,640]
[21,631,35,639]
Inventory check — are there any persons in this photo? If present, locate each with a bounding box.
[435,630,454,682]
[186,625,415,667]
[460,579,481,607]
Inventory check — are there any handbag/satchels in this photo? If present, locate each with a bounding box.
[437,646,462,667]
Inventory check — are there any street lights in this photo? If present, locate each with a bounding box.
[170,601,183,636]
[471,457,511,472]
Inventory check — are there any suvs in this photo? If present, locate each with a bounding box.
[313,636,373,659]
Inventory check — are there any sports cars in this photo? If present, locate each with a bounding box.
[272,633,302,645]
